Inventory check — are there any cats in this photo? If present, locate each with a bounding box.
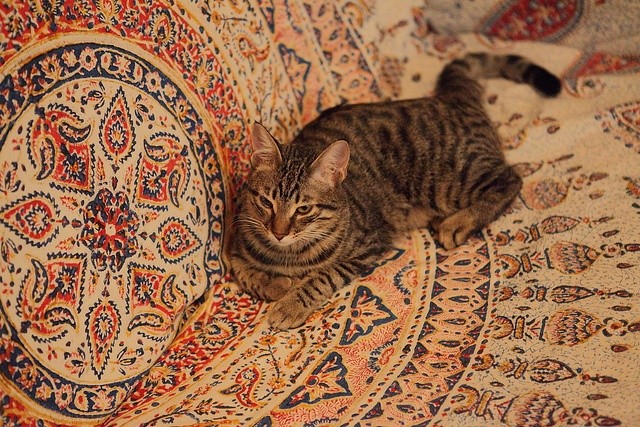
[225,51,562,332]
